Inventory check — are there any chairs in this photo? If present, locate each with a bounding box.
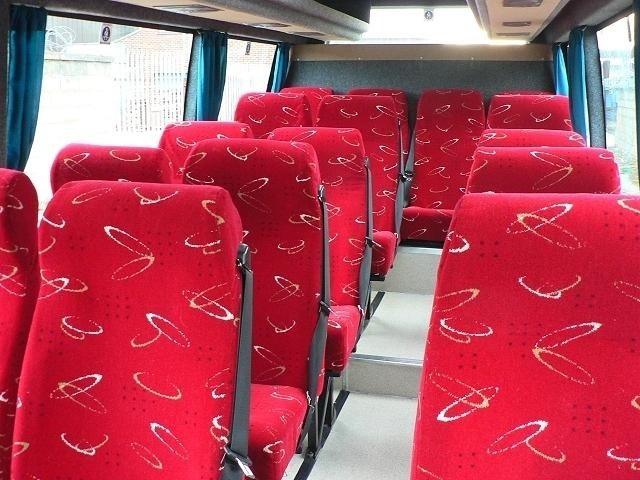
[0,87,640,479]
